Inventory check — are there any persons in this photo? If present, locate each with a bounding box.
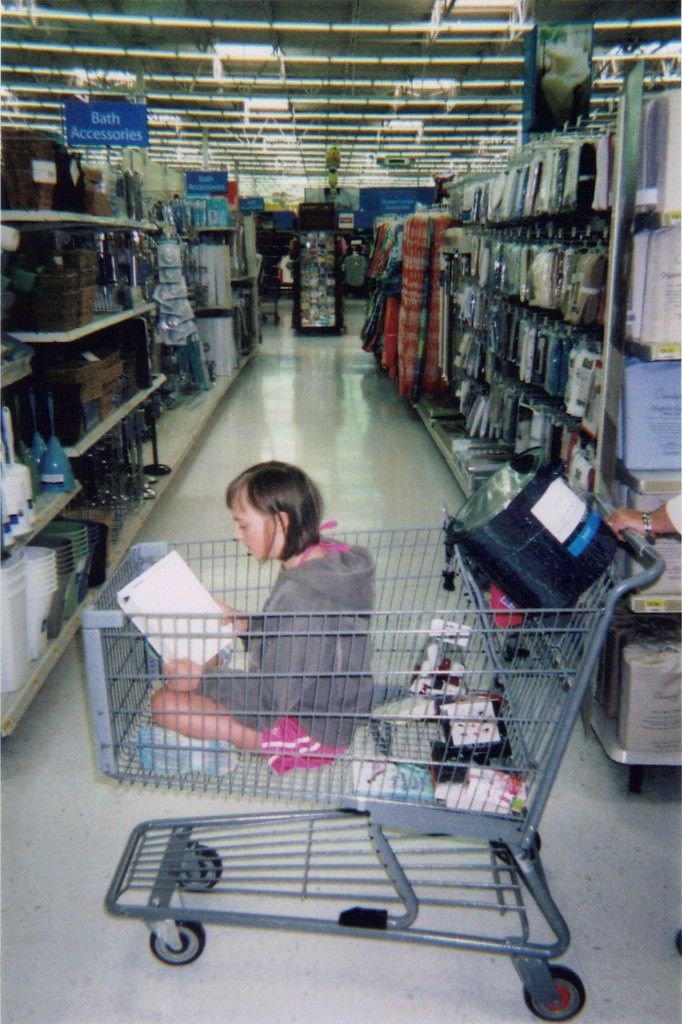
[151,461,378,773]
[605,493,681,954]
[342,245,369,299]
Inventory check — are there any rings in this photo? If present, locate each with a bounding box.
[607,520,614,526]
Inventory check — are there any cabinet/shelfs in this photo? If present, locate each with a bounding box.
[0,211,259,736]
[409,94,681,792]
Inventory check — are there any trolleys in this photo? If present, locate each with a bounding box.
[78,492,666,1022]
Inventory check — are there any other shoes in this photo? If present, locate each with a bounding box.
[137,727,238,777]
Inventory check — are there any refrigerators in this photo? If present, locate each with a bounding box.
[292,202,343,334]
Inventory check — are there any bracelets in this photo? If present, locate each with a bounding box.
[641,511,655,542]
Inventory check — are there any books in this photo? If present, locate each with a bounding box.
[116,549,240,666]
